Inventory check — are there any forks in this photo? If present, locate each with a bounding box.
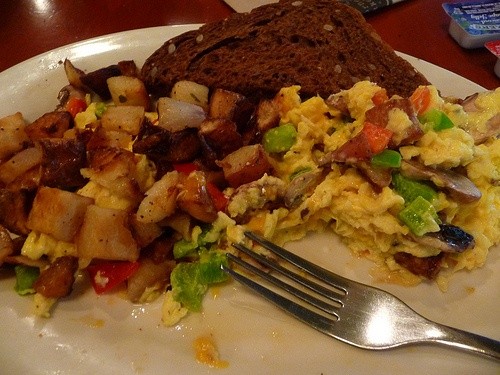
[221,230,500,366]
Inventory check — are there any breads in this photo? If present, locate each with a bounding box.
[140,0,433,99]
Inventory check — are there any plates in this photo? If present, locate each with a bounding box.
[1,25,499,372]
